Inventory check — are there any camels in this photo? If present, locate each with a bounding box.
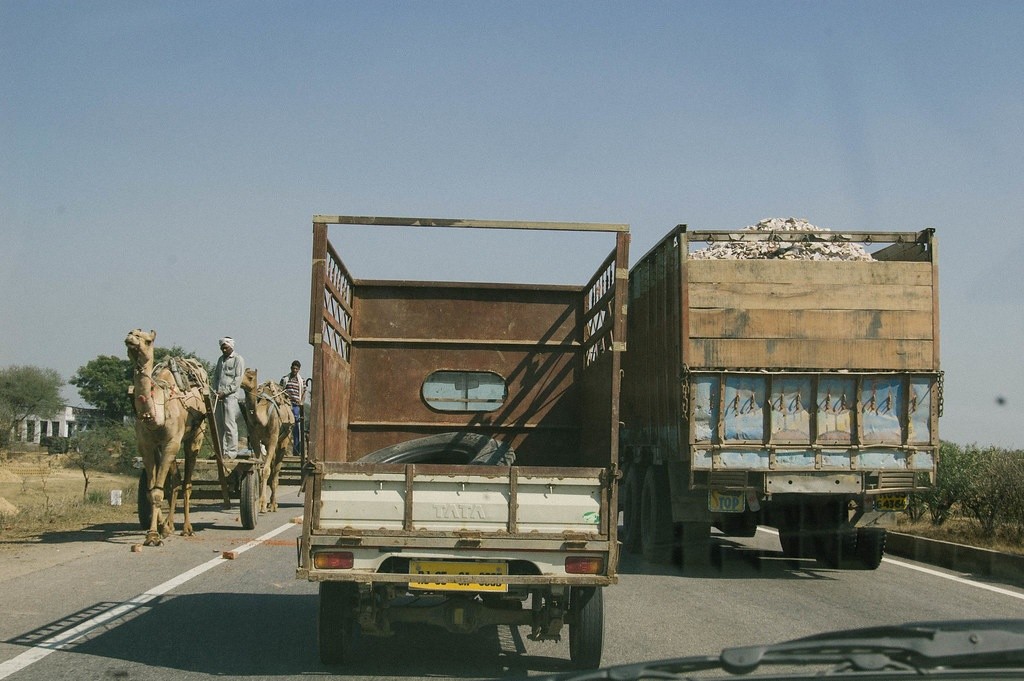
[239,367,294,515]
[124,328,210,548]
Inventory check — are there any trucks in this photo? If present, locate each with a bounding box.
[296,214,631,668]
[625,223,946,572]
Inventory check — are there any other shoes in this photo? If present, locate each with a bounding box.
[293,452,298,456]
[208,454,218,460]
[222,454,230,459]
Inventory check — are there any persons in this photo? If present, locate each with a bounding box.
[206,337,244,459]
[280,360,307,455]
